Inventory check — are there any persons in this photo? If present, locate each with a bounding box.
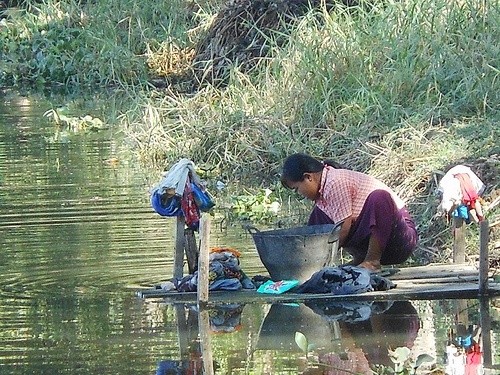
[281,153,418,276]
[303,299,419,375]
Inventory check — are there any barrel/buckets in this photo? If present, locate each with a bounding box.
[248,303,350,361]
[247,220,345,285]
